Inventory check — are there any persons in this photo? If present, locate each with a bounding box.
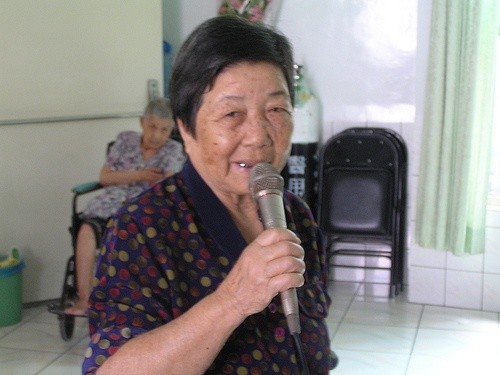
[81,15,339,375]
[63,96,188,314]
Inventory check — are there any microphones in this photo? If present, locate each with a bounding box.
[247,162,301,336]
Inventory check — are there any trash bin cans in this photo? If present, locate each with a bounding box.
[0,245,27,328]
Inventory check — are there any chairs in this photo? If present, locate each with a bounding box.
[317,127,408,298]
